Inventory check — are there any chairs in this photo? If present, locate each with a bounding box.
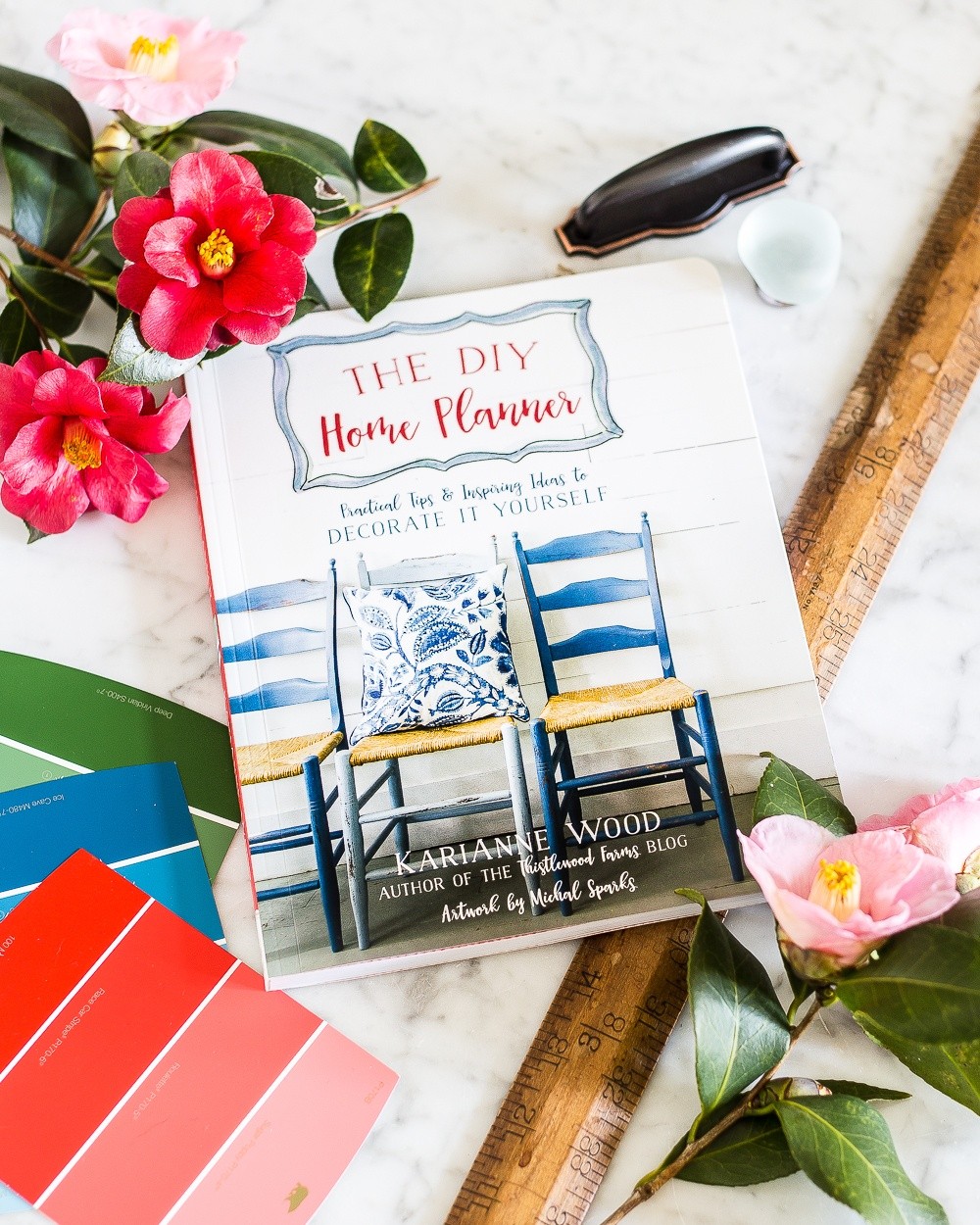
[335,532,542,952]
[212,557,370,954]
[510,514,743,920]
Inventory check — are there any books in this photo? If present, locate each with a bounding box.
[181,257,844,992]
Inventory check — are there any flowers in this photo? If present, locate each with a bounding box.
[0,19,437,546]
[600,749,980,1225]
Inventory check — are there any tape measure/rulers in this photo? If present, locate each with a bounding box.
[441,133,979,1222]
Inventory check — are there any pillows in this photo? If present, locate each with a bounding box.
[349,572,534,745]
